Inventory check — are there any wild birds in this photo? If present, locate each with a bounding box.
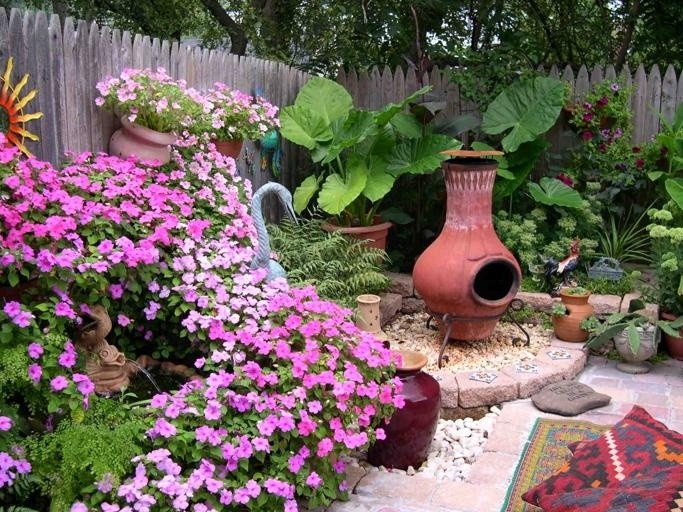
[250,181,300,287]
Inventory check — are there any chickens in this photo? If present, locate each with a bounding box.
[543,240,580,289]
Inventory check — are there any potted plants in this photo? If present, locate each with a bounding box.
[280,76,461,272]
[552,175,683,374]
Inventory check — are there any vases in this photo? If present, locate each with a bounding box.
[209,134,242,163]
[353,293,438,471]
[107,114,174,169]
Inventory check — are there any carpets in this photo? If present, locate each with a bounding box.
[496,406,682,512]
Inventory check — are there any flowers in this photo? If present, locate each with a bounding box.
[93,68,278,142]
